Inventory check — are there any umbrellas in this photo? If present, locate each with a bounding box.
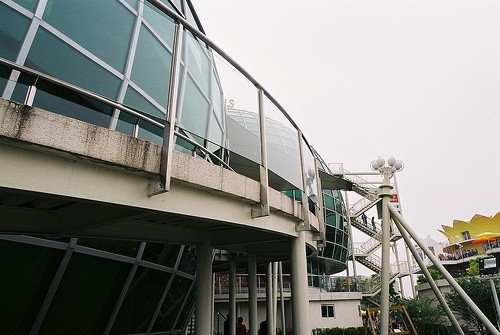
[474,232,499,251]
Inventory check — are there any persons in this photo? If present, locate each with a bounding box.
[236,317,247,335]
[362,213,368,227]
[224,314,229,335]
[392,322,400,330]
[259,320,267,334]
[371,216,376,232]
[438,241,499,260]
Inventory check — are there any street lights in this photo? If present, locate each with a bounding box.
[366,157,406,335]
